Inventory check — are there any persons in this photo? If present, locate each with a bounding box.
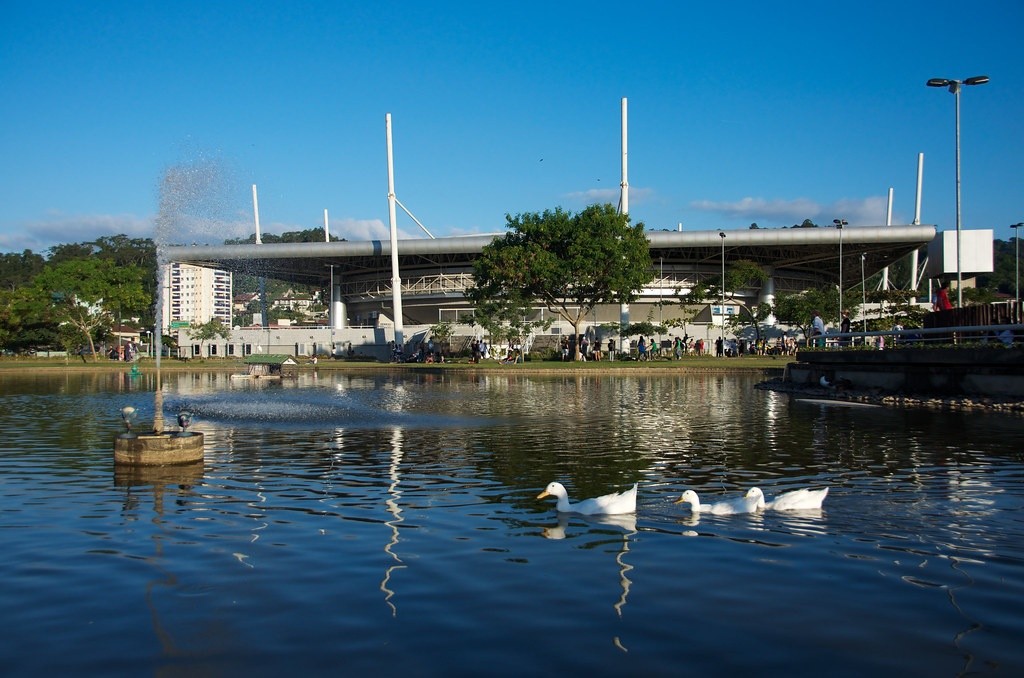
[593,337,602,361]
[672,318,1018,361]
[839,310,852,349]
[933,288,940,312]
[607,339,616,362]
[104,339,143,365]
[648,338,658,361]
[811,309,825,349]
[580,336,589,362]
[561,335,570,362]
[637,336,647,362]
[305,354,317,365]
[329,334,525,364]
[937,284,953,310]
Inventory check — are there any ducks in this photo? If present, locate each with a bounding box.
[536,482,638,515]
[744,486,829,512]
[672,490,761,515]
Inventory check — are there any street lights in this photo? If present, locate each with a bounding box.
[719,231,728,356]
[1009,222,1024,323]
[833,217,848,346]
[927,75,992,308]
